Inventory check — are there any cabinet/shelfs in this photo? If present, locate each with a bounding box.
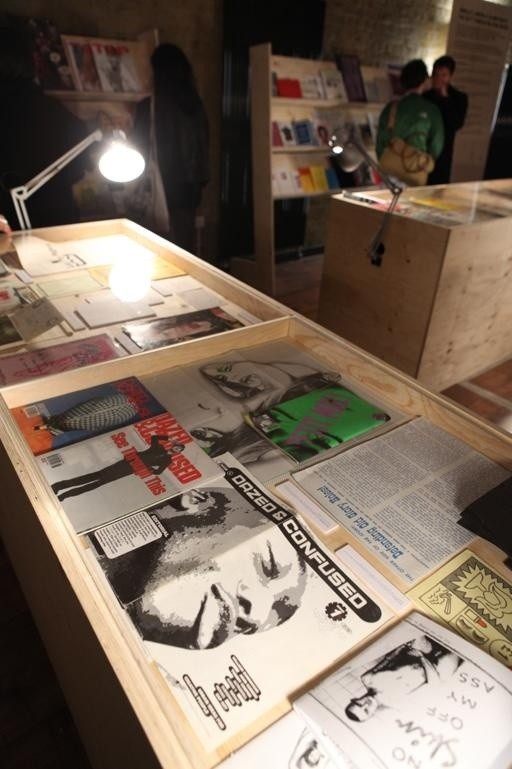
[32,29,169,231]
[219,46,403,262]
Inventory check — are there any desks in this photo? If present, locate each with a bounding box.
[0,217,512,768]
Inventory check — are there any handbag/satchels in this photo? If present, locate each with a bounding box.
[380,137,434,186]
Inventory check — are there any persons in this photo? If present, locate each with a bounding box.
[375,57,446,182]
[345,635,466,723]
[50,434,185,507]
[420,54,469,184]
[132,41,210,252]
[199,360,268,399]
[139,313,226,347]
[98,485,308,650]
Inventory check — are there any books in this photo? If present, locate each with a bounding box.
[272,68,381,192]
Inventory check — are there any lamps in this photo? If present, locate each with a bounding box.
[327,123,406,267]
[9,128,147,230]
